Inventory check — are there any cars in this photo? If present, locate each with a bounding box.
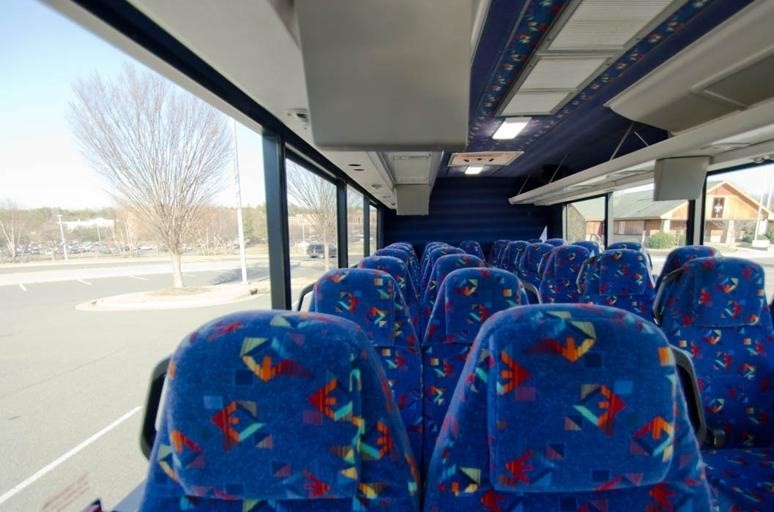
[17,241,127,256]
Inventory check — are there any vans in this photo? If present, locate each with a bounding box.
[306,243,335,258]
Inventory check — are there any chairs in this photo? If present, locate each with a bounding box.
[134,234,772,512]
